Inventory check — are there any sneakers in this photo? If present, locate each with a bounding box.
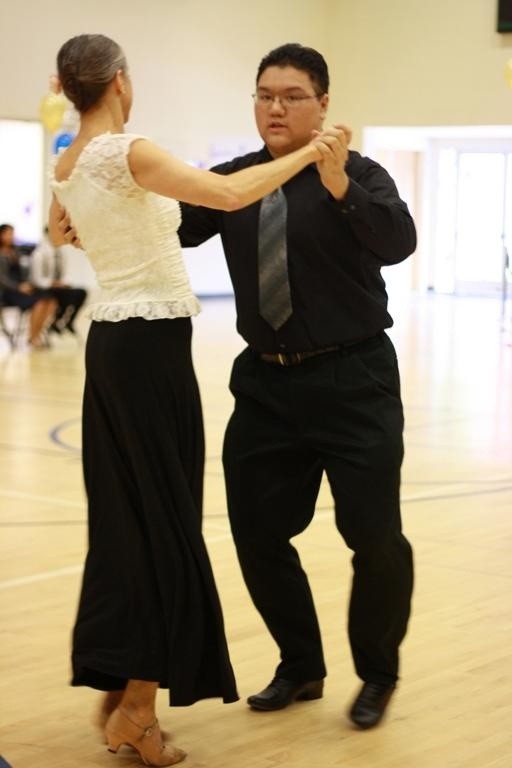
[26,323,77,350]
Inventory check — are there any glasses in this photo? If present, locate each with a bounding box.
[251,92,321,107]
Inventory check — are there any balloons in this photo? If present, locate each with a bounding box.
[54,132,73,155]
[38,91,70,137]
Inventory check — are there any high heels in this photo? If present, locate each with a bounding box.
[92,697,188,768]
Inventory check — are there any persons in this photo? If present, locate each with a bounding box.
[0,224,92,347]
[46,33,353,767]
[47,42,417,730]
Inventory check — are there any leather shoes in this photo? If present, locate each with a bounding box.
[348,680,397,729]
[247,675,326,713]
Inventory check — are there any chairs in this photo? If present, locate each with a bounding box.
[0,303,54,352]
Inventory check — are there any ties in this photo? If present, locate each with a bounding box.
[257,185,293,333]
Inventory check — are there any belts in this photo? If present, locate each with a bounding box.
[261,340,365,368]
